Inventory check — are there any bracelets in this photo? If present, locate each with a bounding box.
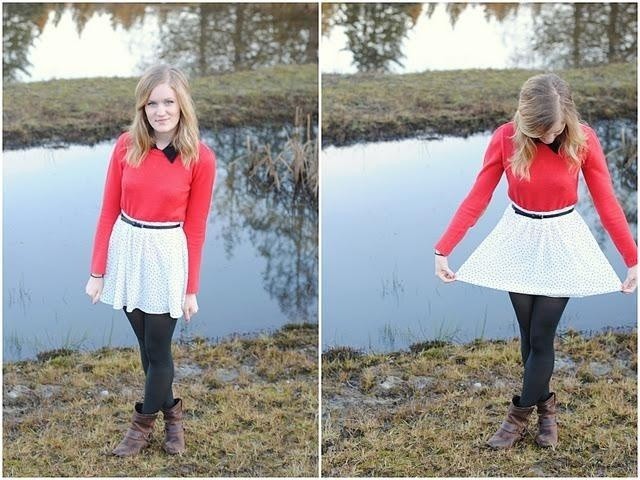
[90,275,102,279]
[435,252,443,257]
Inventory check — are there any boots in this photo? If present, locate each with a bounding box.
[486,395,535,450]
[161,398,185,455]
[112,403,158,457]
[535,393,558,448]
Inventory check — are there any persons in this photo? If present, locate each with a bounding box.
[433,75,638,451]
[85,69,218,457]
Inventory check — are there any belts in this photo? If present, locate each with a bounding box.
[120,214,179,229]
[512,206,574,219]
